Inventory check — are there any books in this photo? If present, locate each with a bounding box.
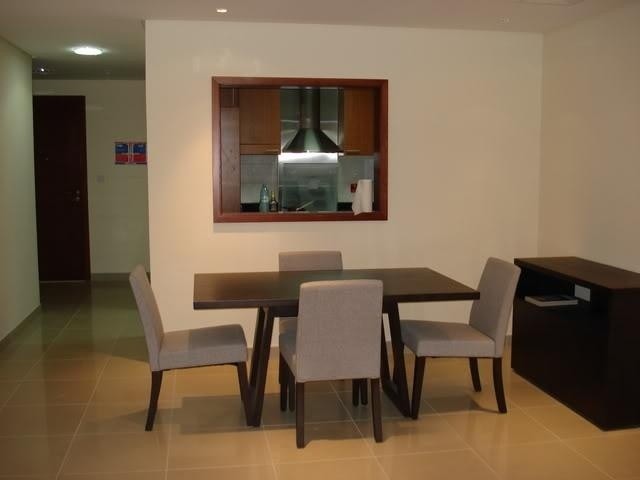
[525,291,578,307]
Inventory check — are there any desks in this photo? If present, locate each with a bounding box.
[193,267,481,427]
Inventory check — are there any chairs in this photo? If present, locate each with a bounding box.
[279,251,369,411]
[129,264,253,432]
[280,279,383,449]
[394,257,522,420]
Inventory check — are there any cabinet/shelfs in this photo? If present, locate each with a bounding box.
[238,87,282,154]
[511,256,640,431]
[340,86,377,156]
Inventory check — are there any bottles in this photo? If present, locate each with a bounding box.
[260,184,278,212]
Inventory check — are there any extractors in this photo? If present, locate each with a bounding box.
[282,87,340,153]
